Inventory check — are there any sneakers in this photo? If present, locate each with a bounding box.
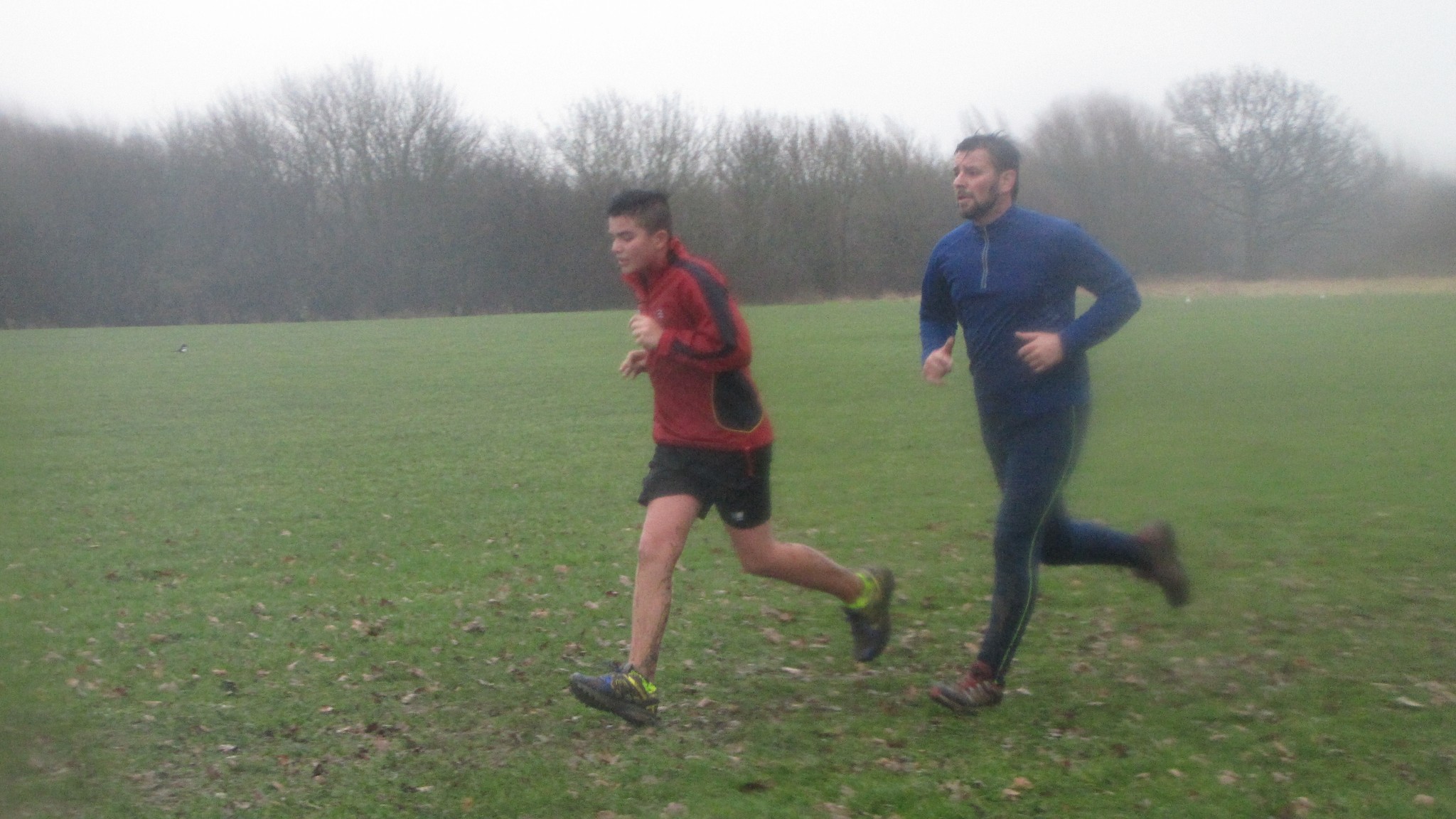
[842,567,894,662]
[570,662,661,725]
[927,660,1005,713]
[1135,518,1190,608]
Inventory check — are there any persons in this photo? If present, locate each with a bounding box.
[565,190,898,727]
[916,131,1190,715]
